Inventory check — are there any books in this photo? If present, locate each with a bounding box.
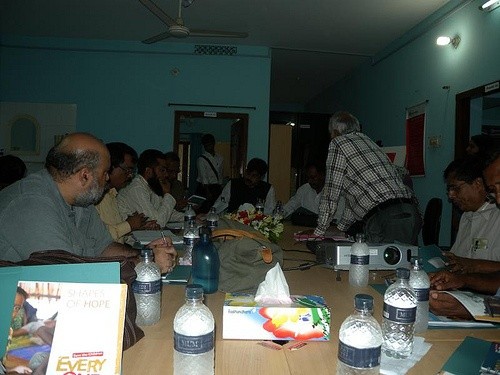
[0,262,128,375]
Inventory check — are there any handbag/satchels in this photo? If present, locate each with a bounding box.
[0,249,143,350]
[210,217,284,296]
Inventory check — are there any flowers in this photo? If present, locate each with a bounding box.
[222,203,287,244]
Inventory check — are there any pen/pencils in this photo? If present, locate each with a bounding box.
[161,231,168,244]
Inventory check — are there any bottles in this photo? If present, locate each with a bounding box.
[133,248,161,324]
[206,207,218,231]
[408,256,430,332]
[184,204,196,230]
[380,267,417,359]
[191,226,220,294]
[273,201,283,216]
[182,220,196,263]
[336,293,383,375]
[255,198,264,214]
[348,233,370,287]
[173,284,215,375]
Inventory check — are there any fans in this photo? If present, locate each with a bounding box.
[138,0,249,44]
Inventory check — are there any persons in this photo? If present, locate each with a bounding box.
[294,112,423,246]
[198,157,500,321]
[0,131,226,275]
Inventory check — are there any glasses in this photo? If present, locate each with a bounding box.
[450,176,475,192]
[118,166,137,178]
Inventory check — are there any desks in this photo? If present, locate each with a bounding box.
[122,226,500,375]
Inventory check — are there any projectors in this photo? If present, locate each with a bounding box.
[315,241,420,270]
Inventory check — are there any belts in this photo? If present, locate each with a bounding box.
[362,198,414,225]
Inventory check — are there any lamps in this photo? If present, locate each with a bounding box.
[479,0,500,13]
[436,33,461,48]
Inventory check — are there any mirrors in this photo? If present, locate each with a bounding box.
[6,112,41,155]
